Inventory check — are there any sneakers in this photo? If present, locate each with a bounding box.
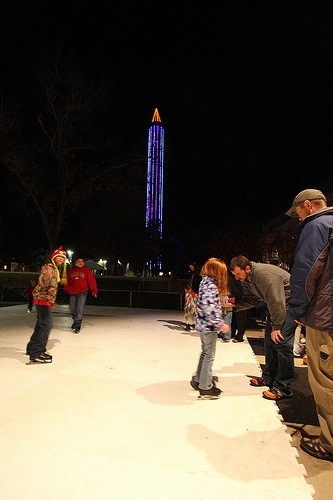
[26,348,48,355]
[29,353,52,364]
[299,435,333,462]
[71,322,81,334]
[190,376,222,400]
[250,377,272,387]
[262,386,293,401]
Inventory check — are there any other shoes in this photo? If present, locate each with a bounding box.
[26,309,31,314]
[221,337,230,342]
[231,335,245,343]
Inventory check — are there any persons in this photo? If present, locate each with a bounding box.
[184,262,202,332]
[228,254,308,401]
[24,278,37,313]
[191,257,231,396]
[223,274,251,342]
[280,189,333,462]
[63,256,98,333]
[26,246,67,363]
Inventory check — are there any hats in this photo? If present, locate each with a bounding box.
[50,246,67,285]
[284,189,327,219]
